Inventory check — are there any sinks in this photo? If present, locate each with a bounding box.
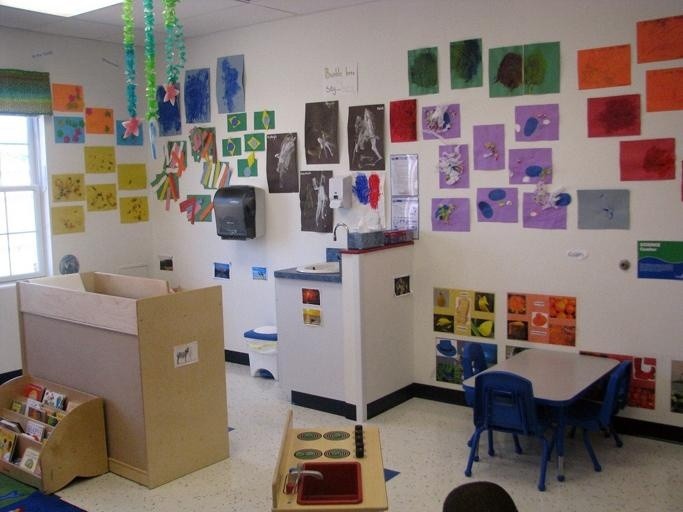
[296,260,340,274]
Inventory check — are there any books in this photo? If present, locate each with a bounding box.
[0,377,83,476]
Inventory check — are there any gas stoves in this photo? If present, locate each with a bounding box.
[287,421,364,464]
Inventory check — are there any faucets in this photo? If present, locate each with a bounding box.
[333,222,350,241]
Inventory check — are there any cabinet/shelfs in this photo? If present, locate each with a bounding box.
[0,375,109,495]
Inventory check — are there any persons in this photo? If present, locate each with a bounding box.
[312,180,329,228]
[352,114,370,165]
[274,133,296,190]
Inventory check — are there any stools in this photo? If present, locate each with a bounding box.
[244,326,278,383]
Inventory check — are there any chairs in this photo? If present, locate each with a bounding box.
[461,343,632,490]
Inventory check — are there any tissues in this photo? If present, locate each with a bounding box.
[348,215,386,249]
